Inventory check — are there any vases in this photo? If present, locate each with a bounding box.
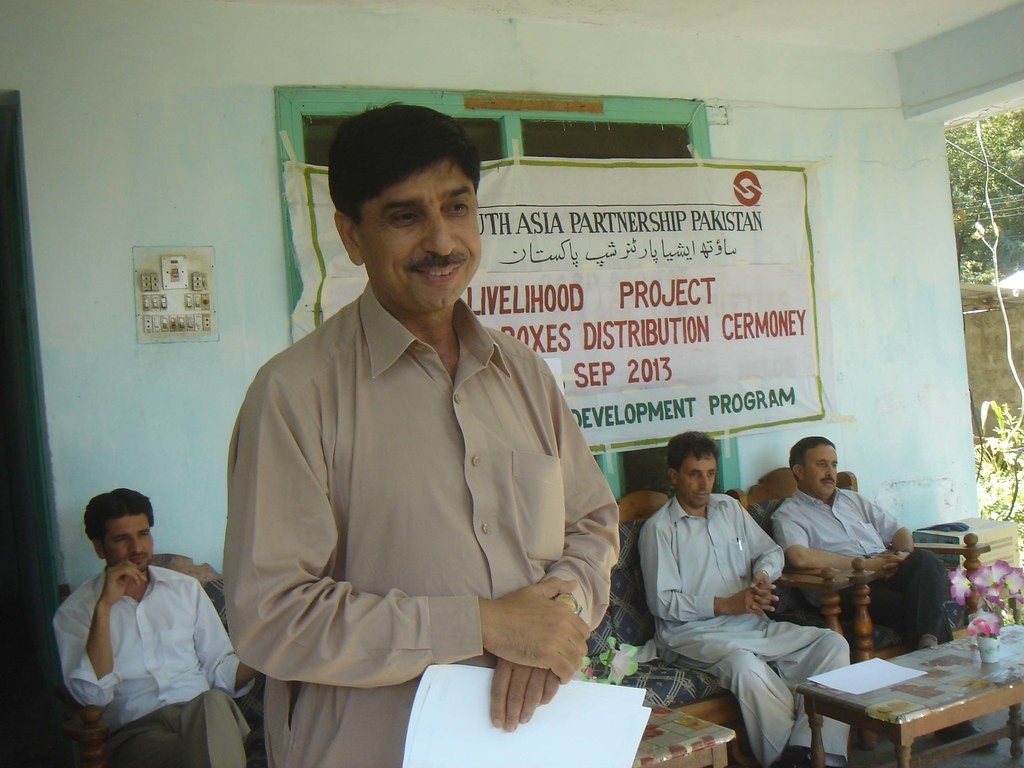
[975,633,1002,664]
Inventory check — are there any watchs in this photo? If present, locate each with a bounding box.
[551,592,582,615]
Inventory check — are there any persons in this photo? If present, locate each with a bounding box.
[639,431,851,768]
[53,488,260,768]
[222,102,621,768]
[771,437,999,752]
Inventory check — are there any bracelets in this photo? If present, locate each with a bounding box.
[895,547,912,555]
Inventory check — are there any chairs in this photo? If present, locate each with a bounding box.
[57,554,266,768]
[726,468,991,662]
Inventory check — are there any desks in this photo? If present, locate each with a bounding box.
[632,699,736,768]
[795,625,1024,768]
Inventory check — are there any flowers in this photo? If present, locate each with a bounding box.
[573,637,659,686]
[948,560,1024,639]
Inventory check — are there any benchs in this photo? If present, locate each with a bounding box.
[585,491,851,764]
